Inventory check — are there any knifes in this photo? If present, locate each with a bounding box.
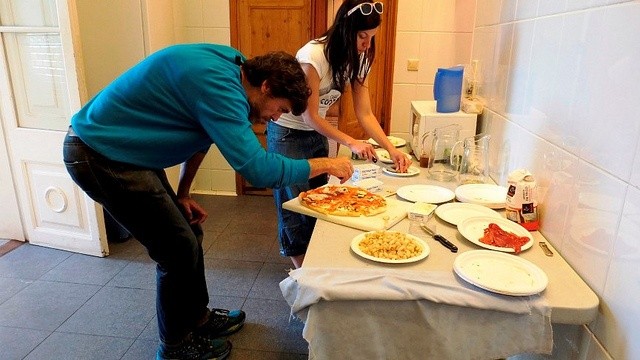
[419,222,458,253]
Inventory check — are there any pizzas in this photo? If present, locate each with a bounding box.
[299,185,387,217]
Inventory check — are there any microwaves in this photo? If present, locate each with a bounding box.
[408,100,477,162]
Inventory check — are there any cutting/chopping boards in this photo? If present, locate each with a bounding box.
[282,184,414,232]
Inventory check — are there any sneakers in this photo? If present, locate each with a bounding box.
[194,307,246,337]
[156,336,232,360]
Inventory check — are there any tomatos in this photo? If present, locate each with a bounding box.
[478,222,531,256]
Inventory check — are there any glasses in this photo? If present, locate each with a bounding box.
[342,1,385,18]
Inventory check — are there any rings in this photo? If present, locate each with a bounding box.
[405,159,408,160]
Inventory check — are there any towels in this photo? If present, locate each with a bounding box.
[279,268,554,358]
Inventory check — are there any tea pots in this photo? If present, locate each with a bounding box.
[420,125,461,182]
[430,65,471,118]
[450,133,491,184]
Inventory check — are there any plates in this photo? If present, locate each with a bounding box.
[382,164,420,176]
[454,250,549,297]
[351,231,430,263]
[435,203,501,226]
[376,150,412,163]
[397,185,455,203]
[455,184,513,209]
[368,136,406,147]
[457,215,534,253]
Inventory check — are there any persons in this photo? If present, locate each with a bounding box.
[267,0,412,269]
[63,43,354,360]
